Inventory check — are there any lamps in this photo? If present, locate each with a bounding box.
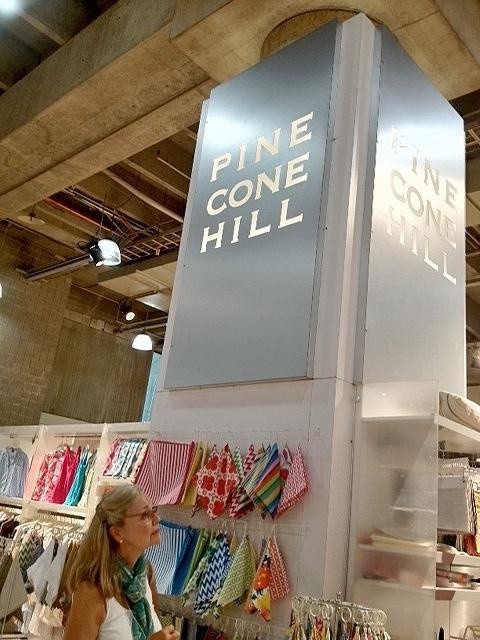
[76,191,123,268]
[131,306,154,350]
[118,296,137,322]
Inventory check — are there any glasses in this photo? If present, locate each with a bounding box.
[126,506,159,523]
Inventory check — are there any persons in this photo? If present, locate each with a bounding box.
[62,482,181,640]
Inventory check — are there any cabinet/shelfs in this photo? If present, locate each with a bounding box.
[343,380,480,640]
[1,423,150,554]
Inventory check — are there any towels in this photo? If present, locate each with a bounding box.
[131,436,309,624]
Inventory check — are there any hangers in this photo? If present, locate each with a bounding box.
[17,510,87,543]
[53,431,101,449]
[112,432,146,444]
[0,504,19,521]
[5,432,18,447]
